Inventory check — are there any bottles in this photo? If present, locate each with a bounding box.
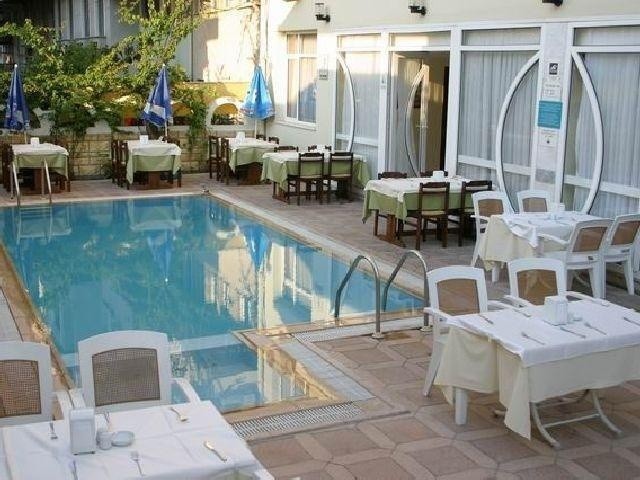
[94,421,115,450]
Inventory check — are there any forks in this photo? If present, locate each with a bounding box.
[130,450,148,477]
[171,406,191,422]
[48,422,59,440]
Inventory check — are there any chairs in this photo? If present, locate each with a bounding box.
[362,166,639,306]
[0,340,73,428]
[109,136,182,189]
[208,131,361,205]
[68,332,200,415]
[1,140,71,193]
[418,266,488,424]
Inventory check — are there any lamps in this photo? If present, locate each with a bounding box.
[408,0,426,16]
[314,3,331,22]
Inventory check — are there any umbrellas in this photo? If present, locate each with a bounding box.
[146,230,171,283]
[242,225,270,274]
[139,62,174,143]
[3,64,30,144]
[239,65,275,138]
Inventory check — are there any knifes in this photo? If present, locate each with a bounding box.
[204,439,228,463]
